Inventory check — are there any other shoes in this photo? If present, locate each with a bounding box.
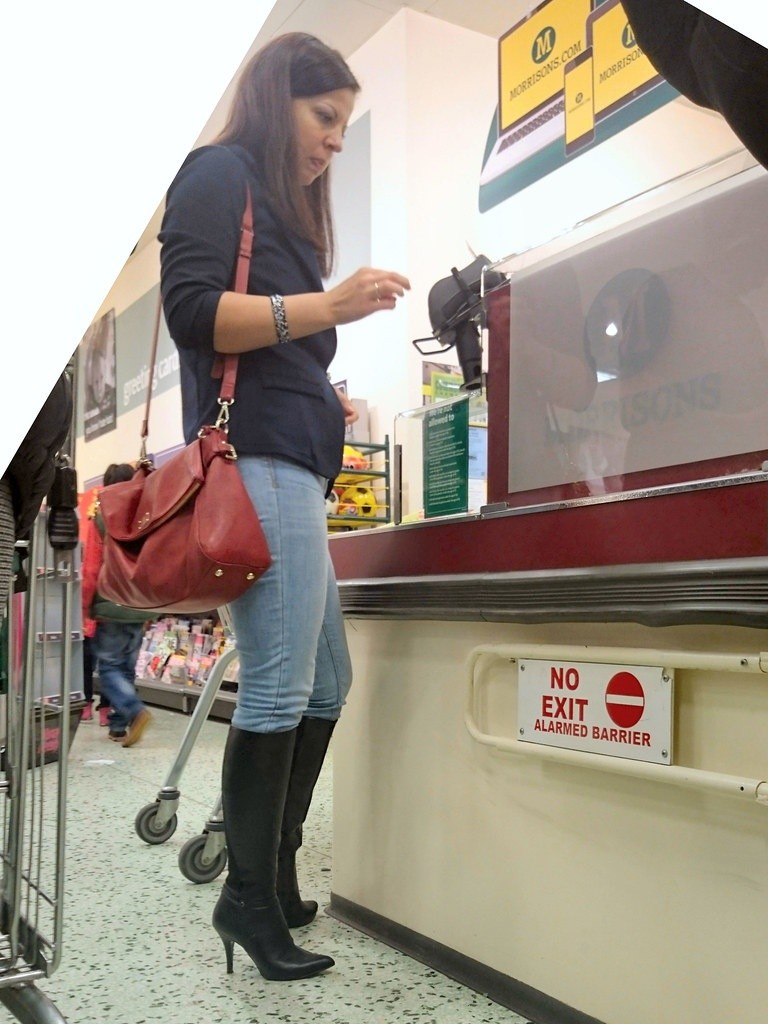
[108,729,128,742]
[122,708,153,747]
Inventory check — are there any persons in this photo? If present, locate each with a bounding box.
[83,350,115,441]
[80,617,114,726]
[154,31,413,979]
[81,463,152,749]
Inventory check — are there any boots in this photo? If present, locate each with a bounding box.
[212,723,336,981]
[275,714,340,930]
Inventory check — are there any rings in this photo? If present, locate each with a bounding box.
[375,282,380,297]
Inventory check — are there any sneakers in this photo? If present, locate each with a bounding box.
[99,706,112,726]
[80,702,93,720]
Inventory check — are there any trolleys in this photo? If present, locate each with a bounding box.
[137,600,235,885]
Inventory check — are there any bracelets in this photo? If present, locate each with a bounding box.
[271,294,290,344]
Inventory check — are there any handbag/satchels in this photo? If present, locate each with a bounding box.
[95,425,273,615]
